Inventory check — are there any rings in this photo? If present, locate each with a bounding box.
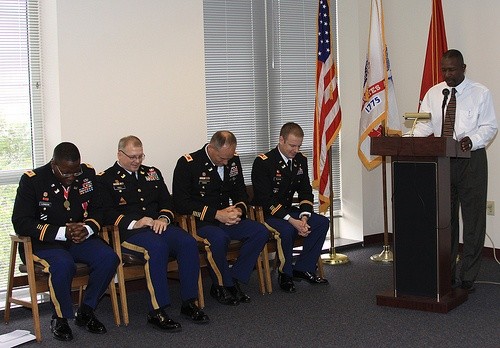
[469,144,471,147]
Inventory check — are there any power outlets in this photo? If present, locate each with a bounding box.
[487,201,495,215]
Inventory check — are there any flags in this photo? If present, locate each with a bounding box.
[358,0,402,171]
[312,0,344,215]
[419,0,447,113]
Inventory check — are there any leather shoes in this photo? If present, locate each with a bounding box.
[209,284,253,306]
[73,308,108,334]
[49,317,73,341]
[277,272,297,294]
[180,303,210,324]
[292,268,329,286]
[146,311,182,333]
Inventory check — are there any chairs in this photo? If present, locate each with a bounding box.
[3,186,326,343]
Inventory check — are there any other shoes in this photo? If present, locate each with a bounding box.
[461,280,476,294]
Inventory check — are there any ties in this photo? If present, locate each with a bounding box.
[131,172,137,183]
[287,160,291,170]
[442,88,458,139]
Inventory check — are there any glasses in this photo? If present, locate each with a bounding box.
[118,150,146,161]
[50,160,83,178]
[440,66,463,72]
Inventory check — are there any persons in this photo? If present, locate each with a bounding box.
[100,135,210,333]
[173,130,269,306]
[251,122,330,291]
[405,49,498,292]
[11,142,120,340]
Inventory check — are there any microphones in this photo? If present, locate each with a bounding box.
[442,88,450,109]
